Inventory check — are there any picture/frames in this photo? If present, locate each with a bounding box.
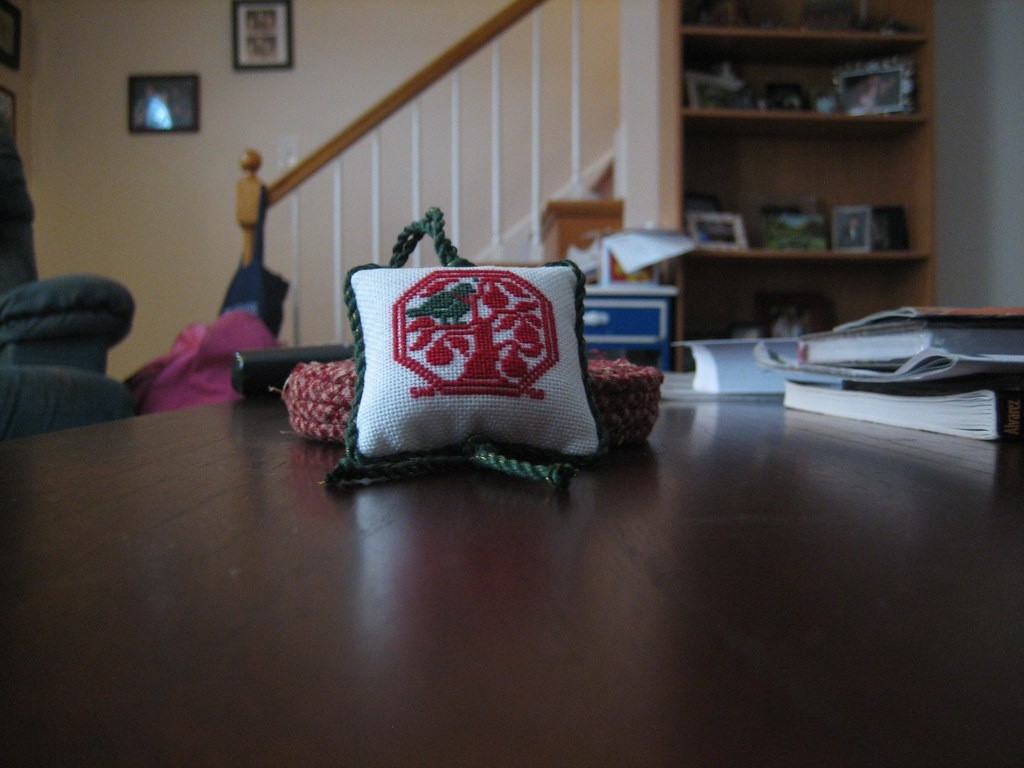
[233,0,293,69]
[686,51,918,341]
[0,85,16,145]
[0,0,22,69]
[127,75,199,133]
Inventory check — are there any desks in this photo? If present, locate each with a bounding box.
[0,398,1024,768]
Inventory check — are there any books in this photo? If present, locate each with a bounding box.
[782,305,1024,442]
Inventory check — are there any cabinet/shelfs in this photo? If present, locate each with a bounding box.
[619,1,936,374]
